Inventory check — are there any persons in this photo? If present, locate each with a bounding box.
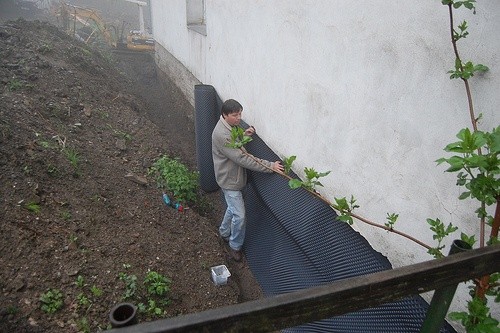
[210,99,286,263]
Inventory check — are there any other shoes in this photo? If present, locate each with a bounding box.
[230,248,241,261]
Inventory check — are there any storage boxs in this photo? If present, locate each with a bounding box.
[211,265,231,286]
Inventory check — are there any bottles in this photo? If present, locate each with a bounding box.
[162,191,170,205]
